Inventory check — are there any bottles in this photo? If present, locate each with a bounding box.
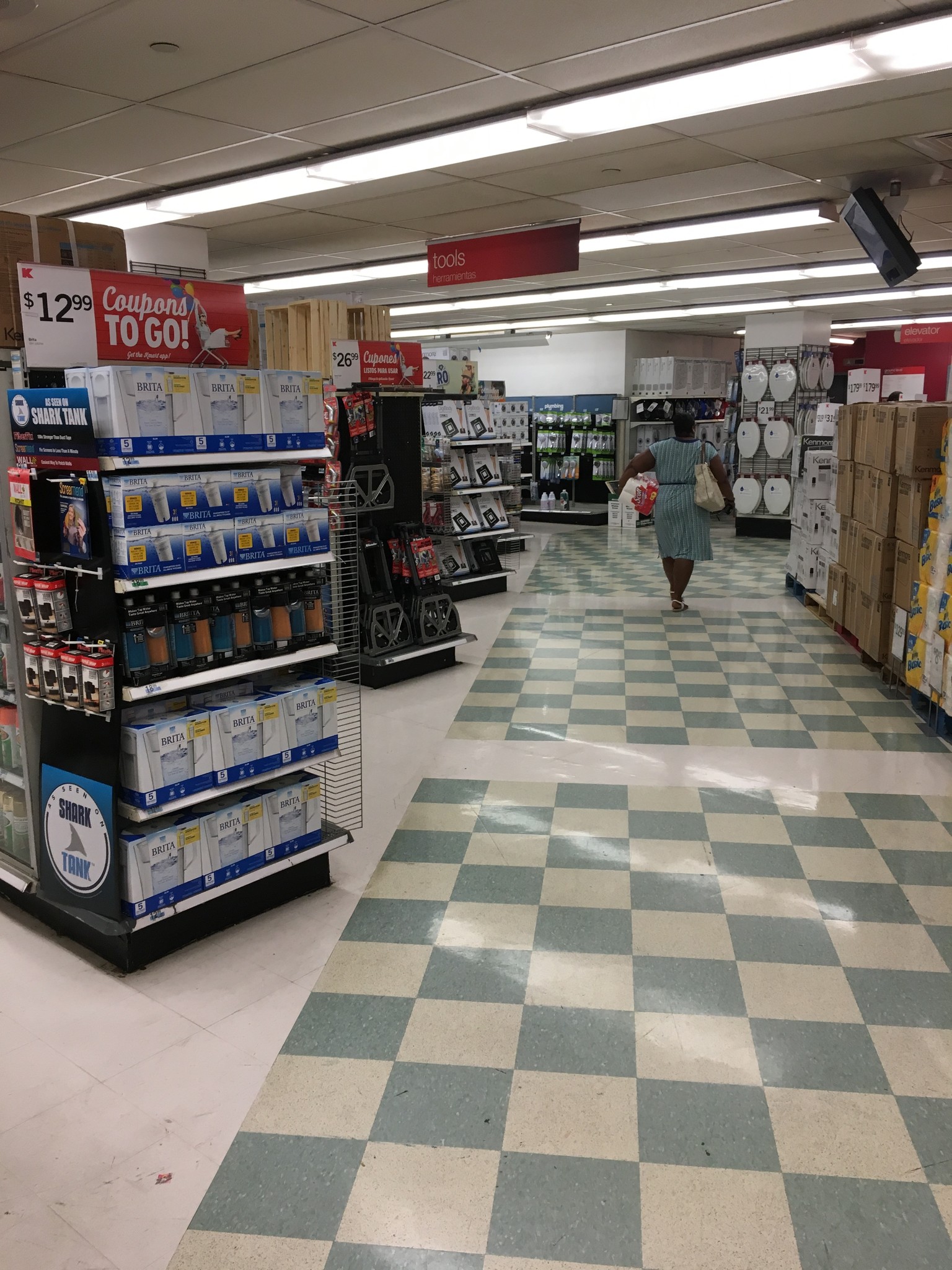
[540,492,549,510]
[549,492,556,509]
[124,570,325,686]
[560,490,570,511]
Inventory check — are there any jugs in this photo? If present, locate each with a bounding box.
[119,368,308,433]
[133,692,325,900]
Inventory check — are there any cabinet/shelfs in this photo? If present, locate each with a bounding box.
[421,357,515,602]
[478,380,535,556]
[626,392,728,523]
[0,261,339,976]
[328,339,480,692]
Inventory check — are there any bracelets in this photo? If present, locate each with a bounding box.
[618,483,625,491]
[724,495,736,502]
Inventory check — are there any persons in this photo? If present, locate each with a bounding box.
[617,411,735,612]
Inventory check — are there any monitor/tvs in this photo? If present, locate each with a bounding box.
[840,186,923,288]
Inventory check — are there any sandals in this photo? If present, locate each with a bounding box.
[672,597,688,612]
[670,591,683,600]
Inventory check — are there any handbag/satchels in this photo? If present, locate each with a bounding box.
[694,441,726,513]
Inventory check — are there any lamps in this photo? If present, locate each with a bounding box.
[61,0,952,344]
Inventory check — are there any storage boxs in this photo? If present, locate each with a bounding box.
[608,492,622,528]
[622,502,656,529]
[784,401,952,743]
[419,397,509,581]
[631,355,731,397]
[0,208,129,350]
[58,362,335,917]
